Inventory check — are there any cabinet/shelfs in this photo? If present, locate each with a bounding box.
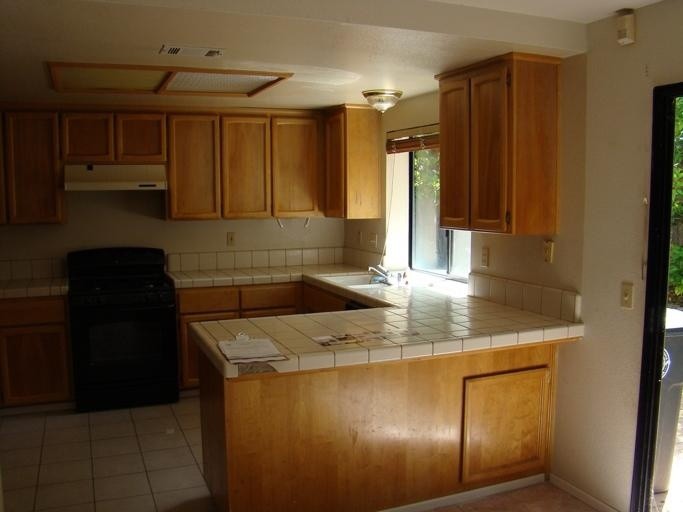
[59,112,167,163]
[176,285,295,390]
[0,295,72,405]
[168,114,221,220]
[325,106,383,220]
[219,114,325,220]
[435,50,558,237]
[0,112,65,224]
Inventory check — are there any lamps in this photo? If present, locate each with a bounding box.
[363,88,403,115]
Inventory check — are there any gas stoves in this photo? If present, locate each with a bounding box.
[66,278,176,309]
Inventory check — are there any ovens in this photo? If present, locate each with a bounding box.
[70,309,180,414]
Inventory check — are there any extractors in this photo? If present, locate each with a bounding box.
[63,164,168,193]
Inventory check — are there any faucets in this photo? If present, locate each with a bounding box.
[368,265,389,277]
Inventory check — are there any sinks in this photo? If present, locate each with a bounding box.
[321,274,391,285]
[348,284,393,288]
